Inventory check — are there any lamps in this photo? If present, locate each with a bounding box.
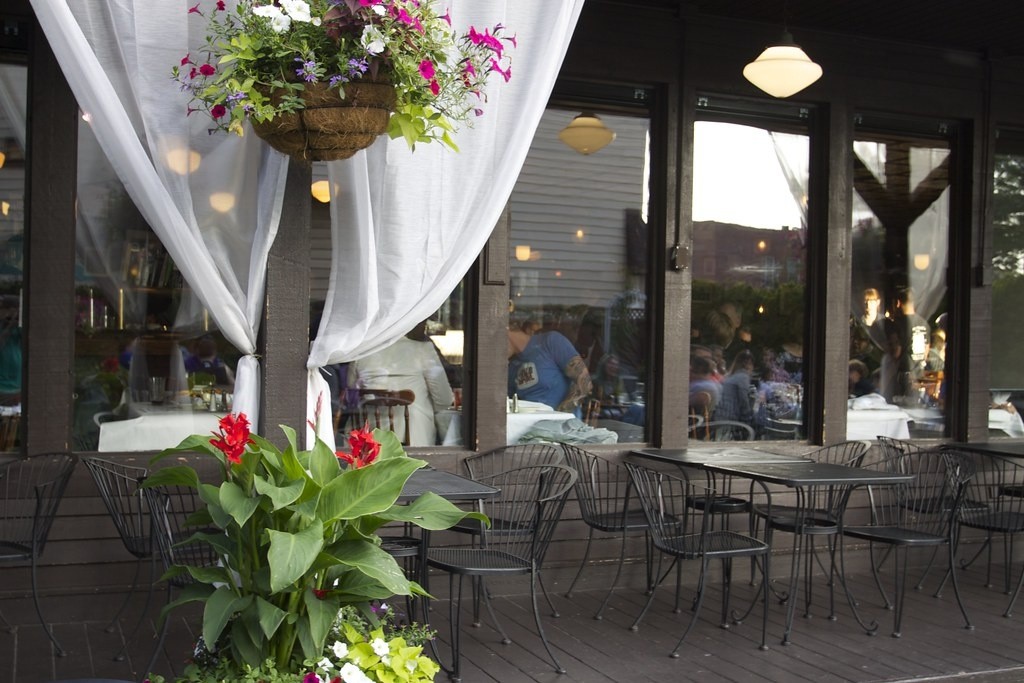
[558,111,617,156]
[743,23,823,98]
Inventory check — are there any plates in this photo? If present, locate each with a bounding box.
[172,396,203,407]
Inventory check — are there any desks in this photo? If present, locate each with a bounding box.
[946,438,1024,569]
[396,470,501,679]
[629,444,812,616]
[706,461,916,645]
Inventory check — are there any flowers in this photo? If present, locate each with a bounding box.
[172,1,517,155]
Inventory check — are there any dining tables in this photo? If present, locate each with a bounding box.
[905,405,1024,436]
[843,396,910,439]
[95,399,229,452]
[430,397,573,447]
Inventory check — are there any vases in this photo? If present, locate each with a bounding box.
[244,70,394,162]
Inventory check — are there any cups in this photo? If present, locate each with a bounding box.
[147,376,166,405]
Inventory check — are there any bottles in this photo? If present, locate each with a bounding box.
[507,396,510,413]
[513,393,518,413]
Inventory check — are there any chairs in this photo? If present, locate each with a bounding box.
[0,369,1024,683]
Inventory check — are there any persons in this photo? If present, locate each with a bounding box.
[508,312,594,420]
[310,299,463,434]
[0,297,22,407]
[523,286,1024,441]
[118,329,235,383]
[344,318,455,448]
